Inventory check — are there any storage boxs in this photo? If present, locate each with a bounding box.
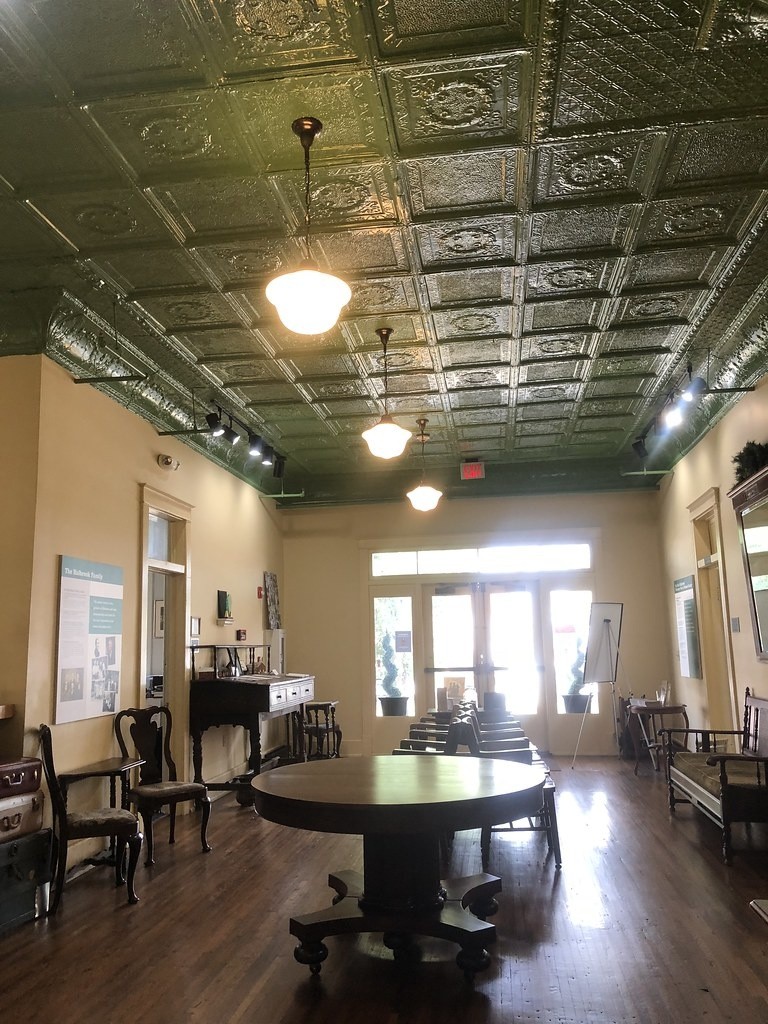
[0,827,52,934]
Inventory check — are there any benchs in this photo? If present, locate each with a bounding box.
[656,687,768,865]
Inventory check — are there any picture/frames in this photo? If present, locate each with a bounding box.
[154,600,165,639]
[191,638,199,653]
[190,616,201,638]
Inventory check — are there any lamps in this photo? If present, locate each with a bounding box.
[205,407,225,437]
[264,117,351,335]
[681,366,707,402]
[221,417,240,445]
[273,459,284,478]
[362,328,412,460]
[262,445,273,465]
[631,438,649,458]
[249,435,262,456]
[406,418,443,511]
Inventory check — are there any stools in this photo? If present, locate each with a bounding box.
[304,723,342,759]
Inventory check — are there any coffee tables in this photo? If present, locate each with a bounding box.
[626,701,690,775]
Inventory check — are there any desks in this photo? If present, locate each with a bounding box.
[57,757,147,877]
[250,753,552,981]
[286,699,341,761]
[189,674,315,809]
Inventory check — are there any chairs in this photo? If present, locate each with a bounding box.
[391,700,562,869]
[39,723,144,917]
[112,705,212,867]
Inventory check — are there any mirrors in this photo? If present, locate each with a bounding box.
[726,491,768,663]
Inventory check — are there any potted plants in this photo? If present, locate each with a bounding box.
[378,629,410,716]
[561,638,594,713]
